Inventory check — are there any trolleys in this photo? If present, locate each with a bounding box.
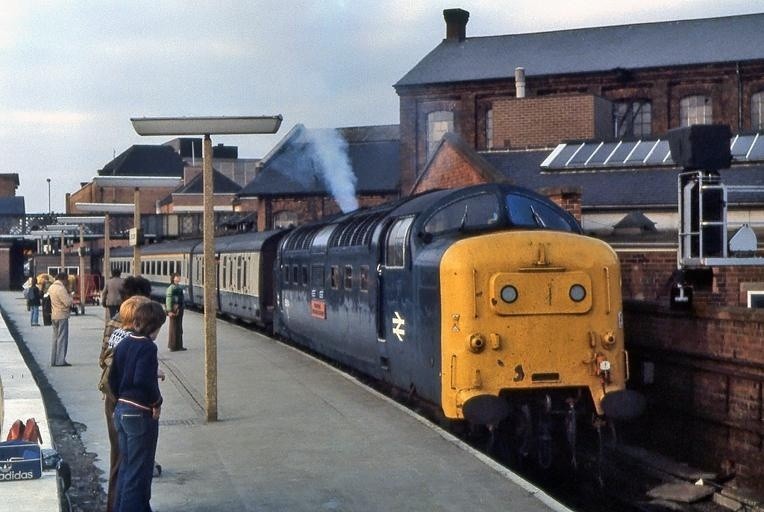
[71,273,101,316]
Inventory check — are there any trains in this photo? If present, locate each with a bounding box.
[90,182,646,456]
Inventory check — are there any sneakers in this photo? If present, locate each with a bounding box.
[170,347,187,352]
[51,363,72,366]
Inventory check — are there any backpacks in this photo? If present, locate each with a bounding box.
[0,440,71,494]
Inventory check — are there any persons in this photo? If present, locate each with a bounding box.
[46,272,74,367]
[24,276,41,327]
[165,272,188,352]
[100,269,125,320]
[106,299,168,512]
[102,293,155,512]
[100,273,164,480]
[38,273,52,326]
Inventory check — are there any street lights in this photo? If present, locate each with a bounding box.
[56,216,106,315]
[46,224,79,272]
[94,176,182,278]
[75,202,135,322]
[131,114,283,421]
[23,229,68,255]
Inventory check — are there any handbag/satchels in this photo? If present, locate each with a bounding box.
[98,353,116,402]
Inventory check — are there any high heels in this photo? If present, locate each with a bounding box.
[6,418,43,444]
